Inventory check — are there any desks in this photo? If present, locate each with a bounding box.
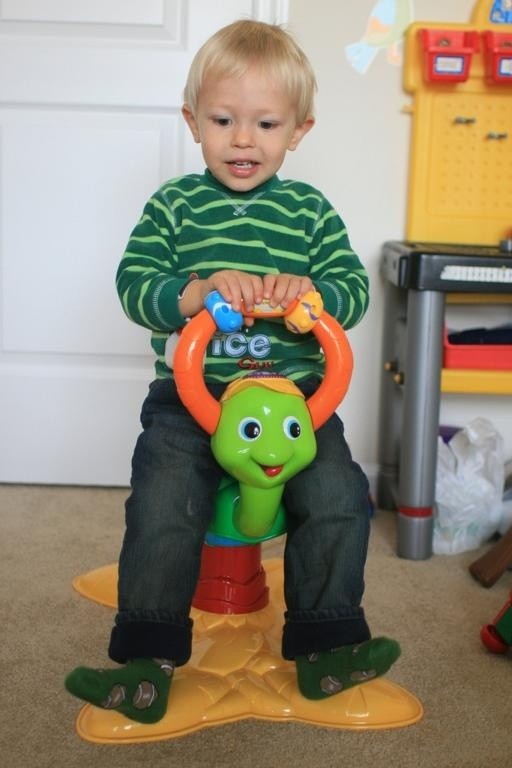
[378,239,512,567]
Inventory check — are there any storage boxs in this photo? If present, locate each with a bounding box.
[440,321,512,372]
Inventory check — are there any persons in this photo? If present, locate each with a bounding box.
[66,19,402,723]
